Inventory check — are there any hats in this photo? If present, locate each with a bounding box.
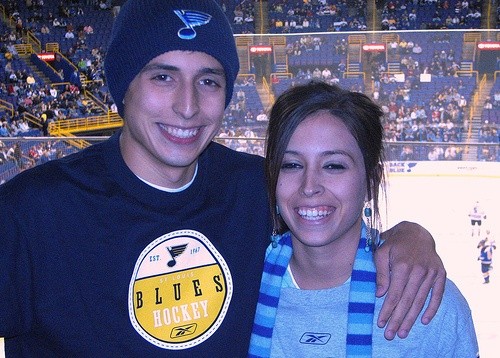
[104,0,239,118]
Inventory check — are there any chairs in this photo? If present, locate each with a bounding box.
[0,0,500,185]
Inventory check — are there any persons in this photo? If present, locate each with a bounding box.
[0,0,447,358]
[0,0,119,137]
[216,78,500,161]
[247,77,480,357]
[220,0,500,32]
[477,230,496,285]
[0,139,77,173]
[468,200,486,238]
[248,35,462,78]
